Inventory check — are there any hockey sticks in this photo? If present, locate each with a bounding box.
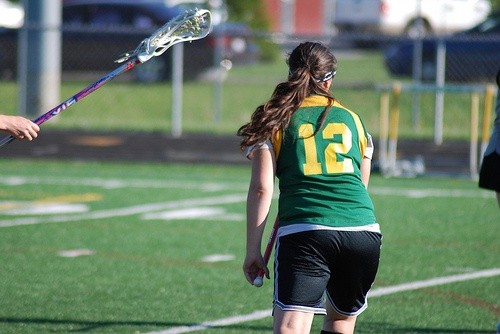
[254,212,278,287]
[0,8,212,149]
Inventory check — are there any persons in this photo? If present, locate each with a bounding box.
[235,39,385,334]
[0,114,41,142]
